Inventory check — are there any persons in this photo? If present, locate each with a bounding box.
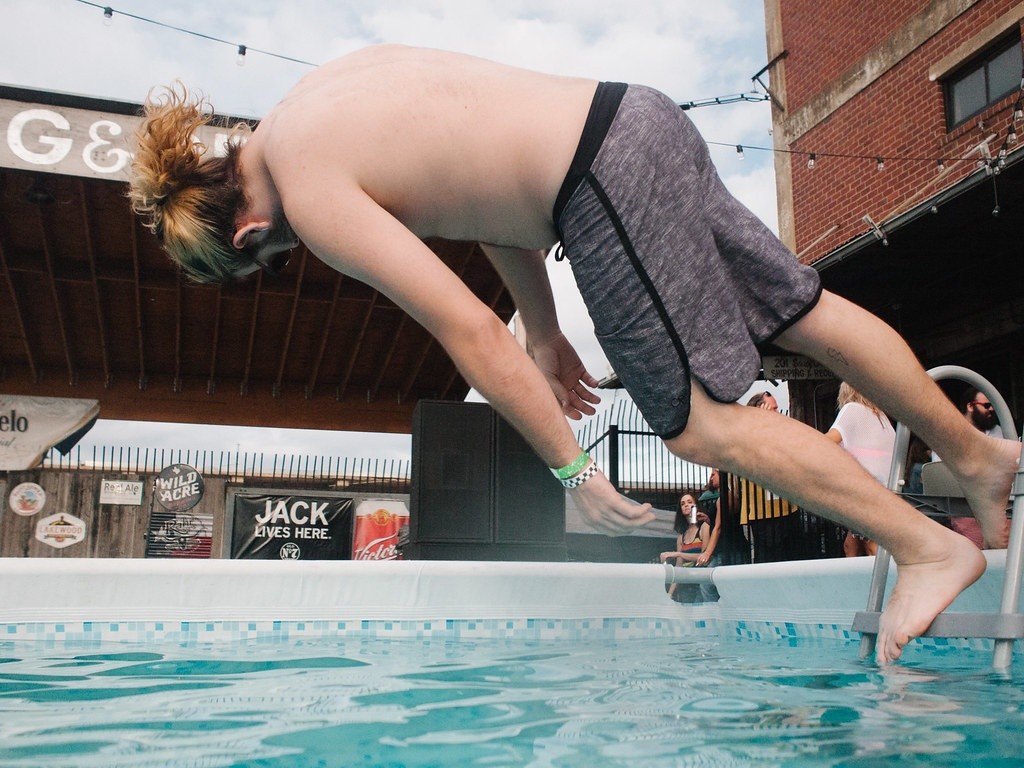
[696,467,750,601]
[823,381,896,558]
[933,388,1012,549]
[902,434,951,531]
[740,391,808,564]
[127,42,1021,666]
[668,492,711,604]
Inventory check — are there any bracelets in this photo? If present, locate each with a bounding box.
[549,447,588,479]
[560,461,598,489]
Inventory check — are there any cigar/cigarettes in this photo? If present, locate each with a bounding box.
[696,563,699,566]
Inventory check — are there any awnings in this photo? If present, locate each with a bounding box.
[799,131,1024,274]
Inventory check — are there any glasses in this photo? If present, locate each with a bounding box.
[228,226,292,277]
[754,390,771,407]
[972,403,992,409]
